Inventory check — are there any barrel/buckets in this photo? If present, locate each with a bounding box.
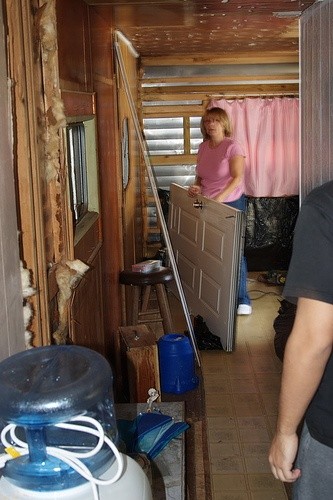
[156,333,199,394]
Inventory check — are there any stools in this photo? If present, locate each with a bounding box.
[121,264,173,336]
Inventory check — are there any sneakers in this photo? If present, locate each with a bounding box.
[237,304,253,315]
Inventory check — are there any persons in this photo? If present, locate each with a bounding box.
[267,179,333,500]
[187,107,252,315]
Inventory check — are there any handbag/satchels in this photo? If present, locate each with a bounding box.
[274,301,297,360]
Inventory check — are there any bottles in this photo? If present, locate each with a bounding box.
[0,345,119,491]
